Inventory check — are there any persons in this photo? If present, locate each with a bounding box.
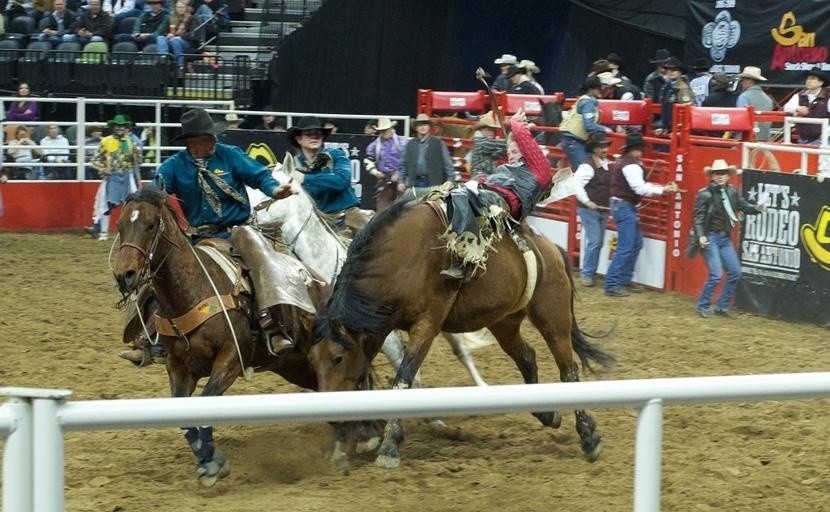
[0,0,259,241]
[119,107,554,365]
[488,49,830,320]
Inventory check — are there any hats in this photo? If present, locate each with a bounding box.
[107,115,131,130]
[801,66,830,87]
[648,49,714,69]
[170,108,245,143]
[410,113,438,132]
[372,118,398,131]
[736,65,768,81]
[474,117,502,129]
[586,131,649,150]
[703,159,737,176]
[270,116,340,147]
[579,52,622,90]
[493,54,541,79]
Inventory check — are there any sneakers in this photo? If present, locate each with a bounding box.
[263,334,294,359]
[84,225,98,239]
[439,262,480,281]
[582,277,645,298]
[118,345,168,365]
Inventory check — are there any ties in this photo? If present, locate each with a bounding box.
[719,188,739,228]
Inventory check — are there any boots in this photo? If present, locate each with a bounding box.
[97,214,111,241]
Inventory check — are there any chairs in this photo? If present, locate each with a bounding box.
[4,124,85,148]
[0,1,205,63]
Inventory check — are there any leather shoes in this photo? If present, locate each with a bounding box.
[699,309,738,320]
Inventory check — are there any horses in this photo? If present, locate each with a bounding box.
[434,112,476,157]
[537,97,566,167]
[112,189,388,489]
[298,195,619,469]
[245,151,500,429]
